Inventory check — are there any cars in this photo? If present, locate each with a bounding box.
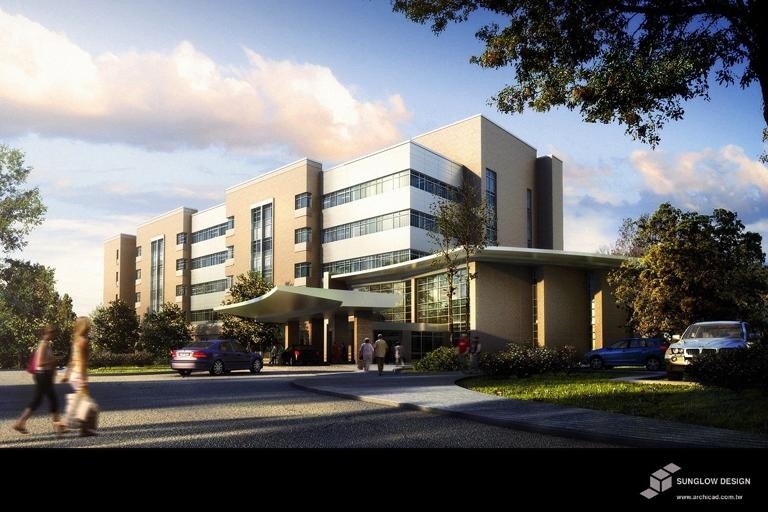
[665,320,760,380]
[171,339,264,376]
[583,337,668,371]
[280,344,320,366]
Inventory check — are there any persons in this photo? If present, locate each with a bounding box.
[469,335,482,370]
[394,340,404,367]
[358,336,376,376]
[327,341,348,363]
[267,342,279,366]
[57,315,103,437]
[455,333,472,373]
[12,327,70,436]
[373,333,389,376]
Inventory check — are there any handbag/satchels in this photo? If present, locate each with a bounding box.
[26,348,38,375]
[60,390,102,431]
